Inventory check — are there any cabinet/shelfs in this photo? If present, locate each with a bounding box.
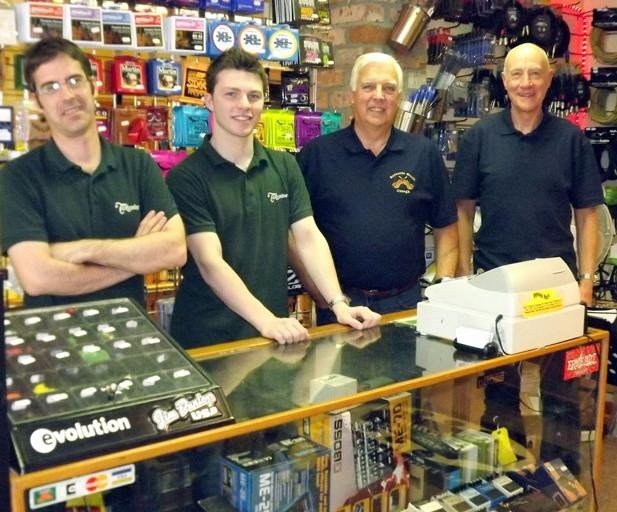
[9,308,610,512]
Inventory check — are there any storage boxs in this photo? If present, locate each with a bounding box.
[218,437,331,512]
[296,378,412,512]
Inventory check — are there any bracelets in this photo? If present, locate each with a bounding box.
[327,294,351,308]
[577,272,601,281]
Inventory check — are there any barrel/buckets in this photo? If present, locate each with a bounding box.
[386,4,431,55]
[394,109,427,136]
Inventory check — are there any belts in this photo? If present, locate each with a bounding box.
[352,273,418,300]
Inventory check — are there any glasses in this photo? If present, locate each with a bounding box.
[41,75,82,94]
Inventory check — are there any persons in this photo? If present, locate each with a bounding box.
[434,40,602,477]
[1,35,189,314]
[161,47,384,353]
[286,51,461,305]
